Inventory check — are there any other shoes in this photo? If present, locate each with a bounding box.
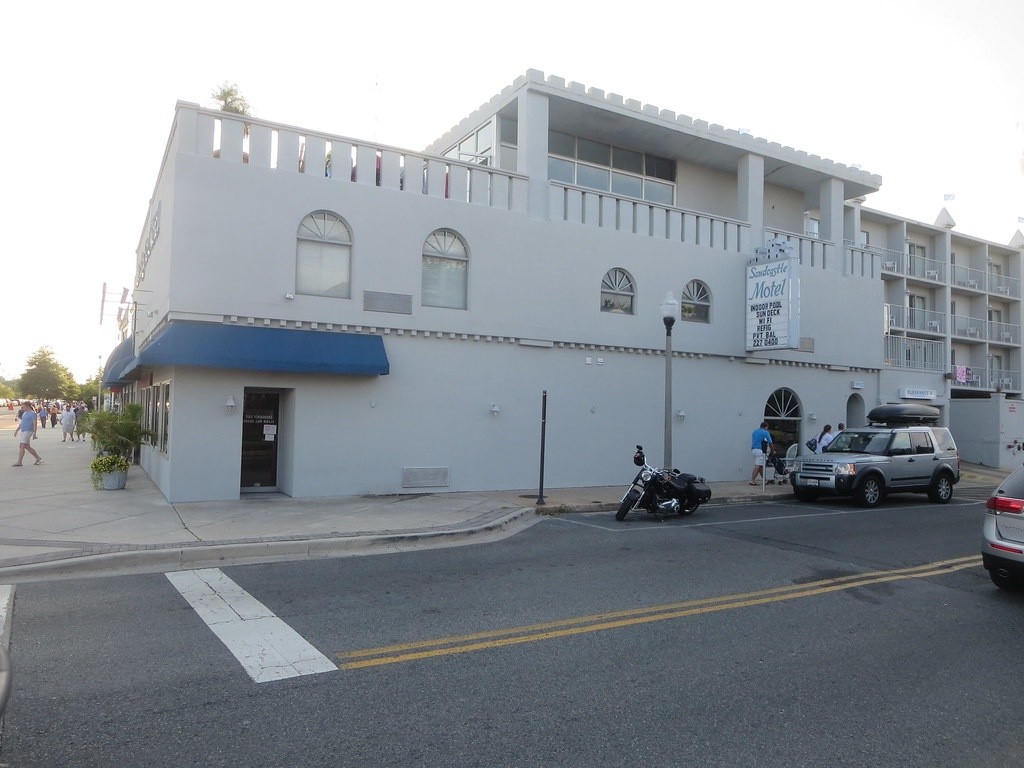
[61,439,85,442]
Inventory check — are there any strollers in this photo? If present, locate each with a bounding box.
[767,453,791,486]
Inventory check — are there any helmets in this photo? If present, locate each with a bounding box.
[634,451,644,466]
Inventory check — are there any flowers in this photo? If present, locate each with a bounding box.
[90,454,129,489]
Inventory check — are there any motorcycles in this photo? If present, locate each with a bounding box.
[615,445,712,521]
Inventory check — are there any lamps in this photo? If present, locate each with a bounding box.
[677,409,685,424]
[224,395,237,415]
[490,402,499,416]
[808,413,817,424]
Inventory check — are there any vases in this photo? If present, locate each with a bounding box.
[101,469,128,490]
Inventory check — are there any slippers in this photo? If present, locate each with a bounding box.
[11,463,23,467]
[750,482,757,485]
[34,458,44,465]
[765,482,770,485]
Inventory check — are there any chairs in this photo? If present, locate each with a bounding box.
[885,261,897,273]
[926,270,939,281]
[968,279,978,288]
[1001,332,1013,343]
[928,321,940,332]
[890,314,895,325]
[967,375,981,388]
[966,327,979,338]
[996,286,1009,295]
[999,378,1012,390]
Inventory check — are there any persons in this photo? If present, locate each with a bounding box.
[59,402,89,442]
[832,423,849,448]
[814,424,834,454]
[50,404,58,427]
[12,401,44,466]
[40,408,48,428]
[749,423,772,485]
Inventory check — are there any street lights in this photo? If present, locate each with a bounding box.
[659,296,679,469]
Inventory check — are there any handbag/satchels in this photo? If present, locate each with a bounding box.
[805,433,819,451]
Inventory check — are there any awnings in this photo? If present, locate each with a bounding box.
[104,335,132,387]
[106,356,136,383]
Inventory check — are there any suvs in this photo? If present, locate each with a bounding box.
[982,463,1024,588]
[790,403,960,508]
